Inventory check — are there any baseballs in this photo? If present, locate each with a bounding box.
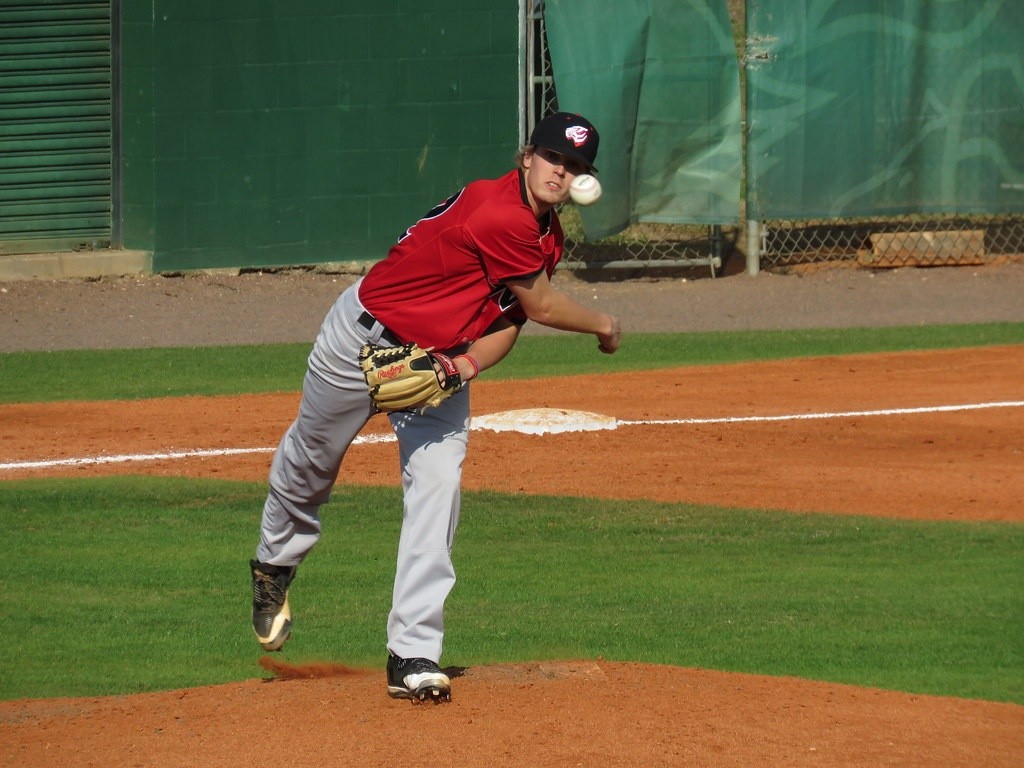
[569,173,603,207]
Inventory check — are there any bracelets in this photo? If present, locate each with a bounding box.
[453,354,479,380]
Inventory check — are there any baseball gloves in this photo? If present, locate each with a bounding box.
[357,338,467,419]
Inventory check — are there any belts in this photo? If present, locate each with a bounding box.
[357,311,404,347]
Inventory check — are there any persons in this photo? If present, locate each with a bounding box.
[249,112,623,697]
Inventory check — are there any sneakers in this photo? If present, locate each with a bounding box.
[387,655,451,702]
[250,558,296,652]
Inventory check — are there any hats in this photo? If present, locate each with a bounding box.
[530,112,600,173]
